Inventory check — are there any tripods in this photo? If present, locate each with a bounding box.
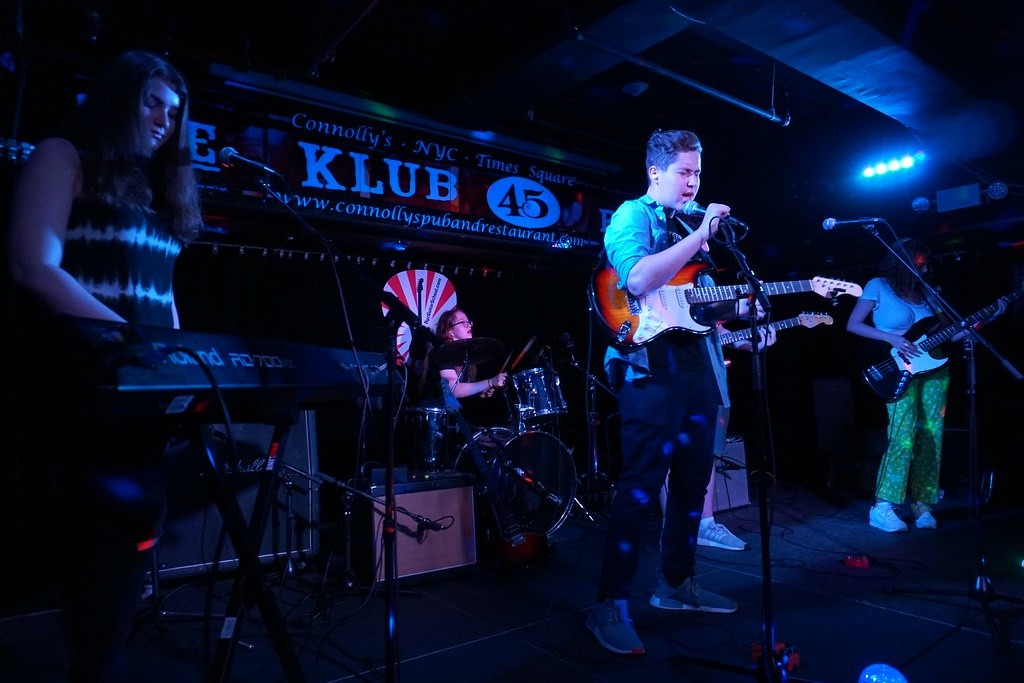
[862,223,1024,652]
[667,224,827,683]
[568,349,620,503]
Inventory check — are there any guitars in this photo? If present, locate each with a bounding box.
[584,257,865,353]
[718,310,834,347]
[858,282,1024,403]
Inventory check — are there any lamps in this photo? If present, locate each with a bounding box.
[911,181,1008,215]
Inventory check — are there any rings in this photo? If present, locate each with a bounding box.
[905,343,909,346]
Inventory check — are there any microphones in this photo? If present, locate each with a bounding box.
[534,347,549,364]
[683,199,748,230]
[822,217,885,231]
[721,466,746,470]
[546,494,562,505]
[418,517,446,531]
[219,147,285,182]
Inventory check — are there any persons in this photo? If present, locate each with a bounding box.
[11,51,205,682]
[583,131,776,653]
[436,308,509,397]
[846,239,1008,532]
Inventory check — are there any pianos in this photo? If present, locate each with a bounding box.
[52,309,416,683]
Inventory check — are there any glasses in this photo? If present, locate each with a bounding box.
[451,321,474,328]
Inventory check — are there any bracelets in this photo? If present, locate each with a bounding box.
[488,379,491,388]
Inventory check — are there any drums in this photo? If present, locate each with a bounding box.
[500,363,570,432]
[396,403,458,470]
[451,426,578,538]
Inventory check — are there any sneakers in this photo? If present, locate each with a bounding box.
[906,500,937,529]
[586,599,645,655]
[649,574,739,613]
[870,502,908,533]
[697,521,749,551]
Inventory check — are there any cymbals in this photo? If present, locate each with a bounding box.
[428,336,504,365]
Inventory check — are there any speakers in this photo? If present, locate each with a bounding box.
[159,407,320,581]
[711,434,749,512]
[347,471,478,588]
